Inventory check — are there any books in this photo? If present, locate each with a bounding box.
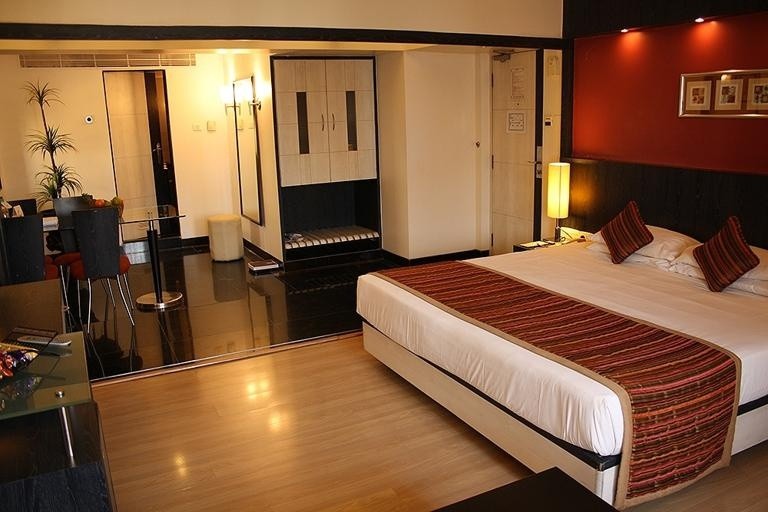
[247,255,279,274]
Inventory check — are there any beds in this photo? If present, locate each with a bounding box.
[352,201,767,506]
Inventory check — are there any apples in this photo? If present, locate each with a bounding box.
[95,200,105,207]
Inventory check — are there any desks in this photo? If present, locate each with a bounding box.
[429,462,615,510]
[5,204,190,313]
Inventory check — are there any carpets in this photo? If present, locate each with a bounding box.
[268,249,409,295]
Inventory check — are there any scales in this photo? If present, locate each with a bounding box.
[248,259,279,271]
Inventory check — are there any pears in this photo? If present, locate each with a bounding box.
[113,197,122,206]
[105,201,111,206]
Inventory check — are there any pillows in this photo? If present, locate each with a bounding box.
[595,199,654,264]
[667,261,767,299]
[583,223,698,264]
[673,239,768,282]
[691,214,761,292]
[587,234,681,273]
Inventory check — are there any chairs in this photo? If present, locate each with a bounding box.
[1,211,48,286]
[0,274,68,340]
[51,195,118,324]
[1,197,38,216]
[73,204,136,332]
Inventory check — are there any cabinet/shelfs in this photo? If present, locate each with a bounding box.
[0,331,117,511]
[268,52,385,291]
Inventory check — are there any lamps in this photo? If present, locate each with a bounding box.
[226,89,247,114]
[241,84,267,116]
[546,161,572,245]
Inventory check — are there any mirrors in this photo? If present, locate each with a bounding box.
[102,69,179,244]
[228,73,266,230]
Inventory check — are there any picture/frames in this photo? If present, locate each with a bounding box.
[677,68,768,123]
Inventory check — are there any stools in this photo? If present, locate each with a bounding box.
[205,214,245,263]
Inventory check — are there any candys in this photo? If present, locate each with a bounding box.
[0,378,32,411]
[0,346,32,383]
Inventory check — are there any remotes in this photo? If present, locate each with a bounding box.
[17,335,72,347]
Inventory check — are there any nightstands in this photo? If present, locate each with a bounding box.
[511,237,573,250]
